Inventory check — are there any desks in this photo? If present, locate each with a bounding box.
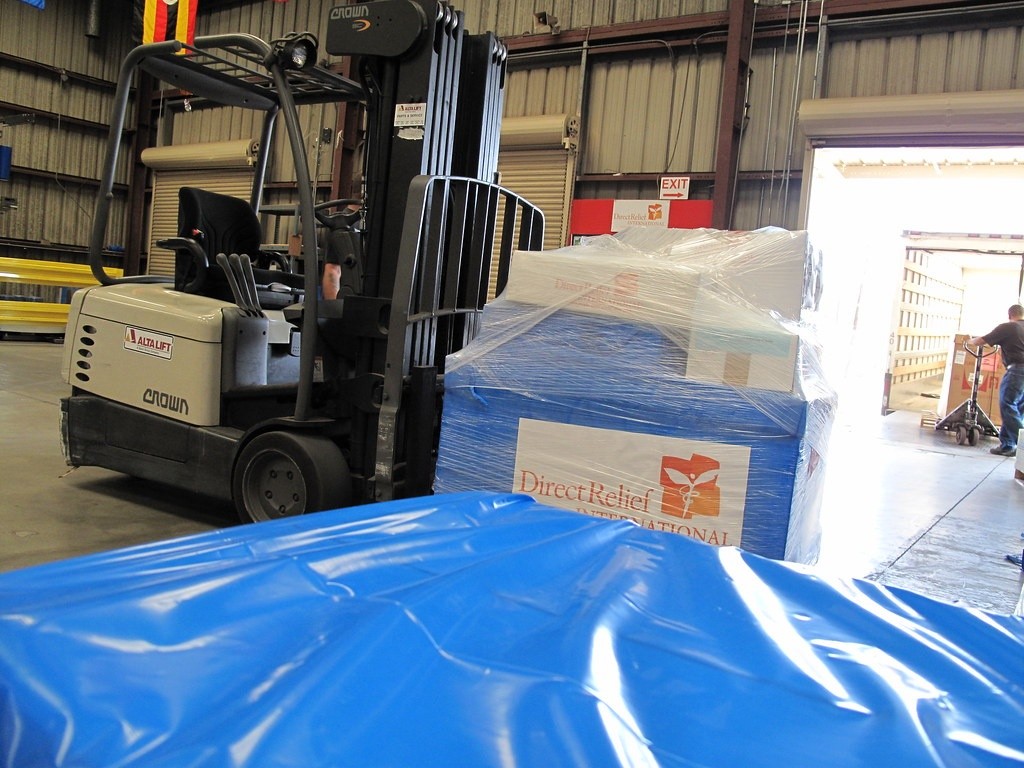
[0,489,1024,768]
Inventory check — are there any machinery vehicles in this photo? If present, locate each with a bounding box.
[55,1,549,525]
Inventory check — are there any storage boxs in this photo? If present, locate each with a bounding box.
[624,225,809,322]
[506,249,701,331]
[990,347,1007,427]
[686,316,800,393]
[936,334,996,419]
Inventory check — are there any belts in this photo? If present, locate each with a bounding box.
[1006,363,1024,369]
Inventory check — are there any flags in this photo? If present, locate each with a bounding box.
[142,0,198,56]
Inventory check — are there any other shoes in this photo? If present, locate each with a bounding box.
[1015,470,1024,480]
[990,445,1017,456]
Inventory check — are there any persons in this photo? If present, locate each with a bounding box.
[972,304,1023,456]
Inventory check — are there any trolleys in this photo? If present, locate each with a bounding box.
[931,336,1001,446]
